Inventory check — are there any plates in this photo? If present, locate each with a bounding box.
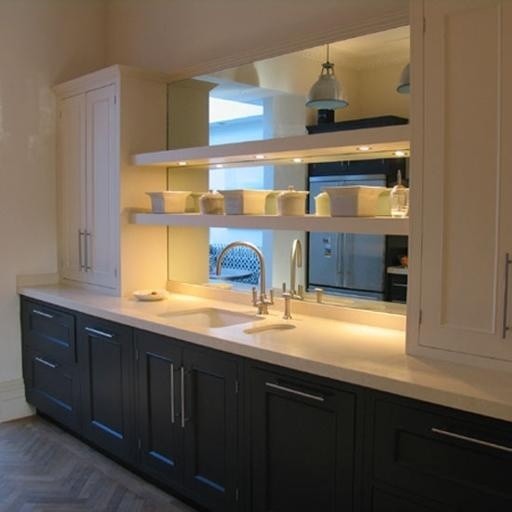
[133,292,163,301]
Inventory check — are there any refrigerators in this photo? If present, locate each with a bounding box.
[309,174,388,302]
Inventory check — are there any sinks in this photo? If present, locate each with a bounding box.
[156,307,266,329]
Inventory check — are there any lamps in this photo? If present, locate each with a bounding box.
[305,42,349,111]
[396,63,410,95]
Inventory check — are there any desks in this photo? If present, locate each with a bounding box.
[209,269,253,281]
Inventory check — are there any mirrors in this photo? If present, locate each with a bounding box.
[166,21,410,331]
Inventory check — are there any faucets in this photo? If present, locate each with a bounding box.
[282,239,304,300]
[216,242,274,314]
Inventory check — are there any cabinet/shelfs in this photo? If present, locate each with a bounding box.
[133,125,409,236]
[19,295,76,437]
[369,388,512,512]
[406,0,512,372]
[49,63,132,296]
[135,323,242,512]
[76,310,134,475]
[242,355,369,512]
